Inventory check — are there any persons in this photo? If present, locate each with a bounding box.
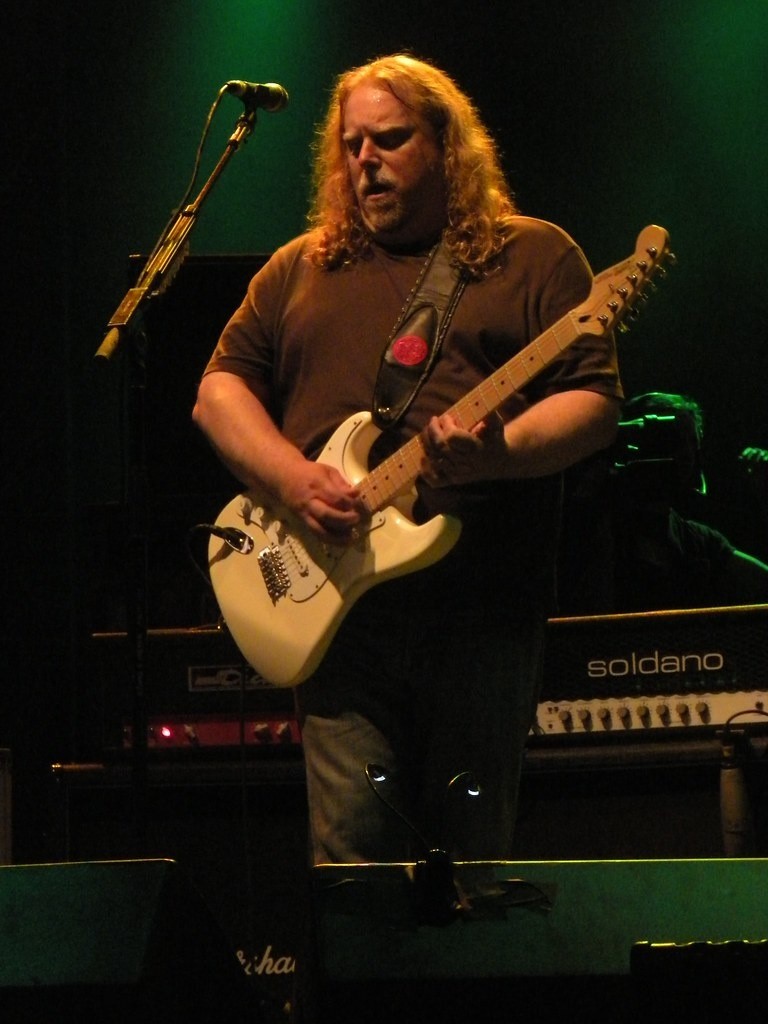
[182,44,624,867]
[594,389,768,618]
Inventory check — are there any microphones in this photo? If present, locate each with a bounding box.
[227,80,290,112]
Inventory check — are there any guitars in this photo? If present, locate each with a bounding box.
[209,224,676,688]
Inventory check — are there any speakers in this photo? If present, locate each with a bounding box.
[2,858,265,1023]
